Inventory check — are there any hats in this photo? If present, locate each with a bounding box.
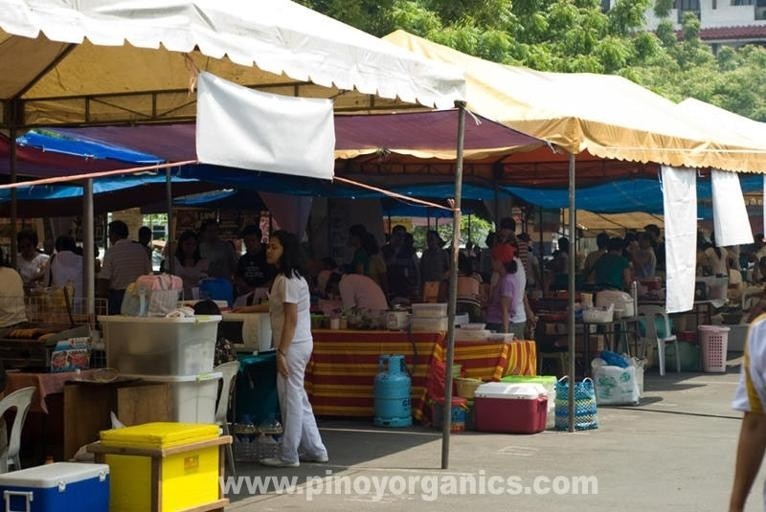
[490,243,517,263]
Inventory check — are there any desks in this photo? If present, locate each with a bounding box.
[303,328,538,429]
[536,317,636,382]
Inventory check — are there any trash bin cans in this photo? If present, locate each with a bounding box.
[698,324,731,374]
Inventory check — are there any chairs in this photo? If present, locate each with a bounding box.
[636,304,681,377]
[0,385,36,474]
[213,360,241,476]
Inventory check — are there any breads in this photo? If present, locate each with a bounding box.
[9,328,57,342]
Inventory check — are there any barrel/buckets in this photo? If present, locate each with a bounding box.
[437,396,471,431]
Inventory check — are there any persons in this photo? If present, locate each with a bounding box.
[0,215,766,344]
[727,311,766,512]
[266,231,330,469]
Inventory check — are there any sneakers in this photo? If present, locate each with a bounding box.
[299,453,328,462]
[260,457,300,467]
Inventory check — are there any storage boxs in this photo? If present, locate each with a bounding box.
[474,382,548,434]
[728,325,748,351]
[96,314,223,377]
[0,462,111,512]
[709,276,729,299]
[99,422,220,512]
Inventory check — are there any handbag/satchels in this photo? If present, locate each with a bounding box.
[121,273,183,315]
[555,375,598,431]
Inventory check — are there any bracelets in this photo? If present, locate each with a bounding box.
[277,347,285,356]
